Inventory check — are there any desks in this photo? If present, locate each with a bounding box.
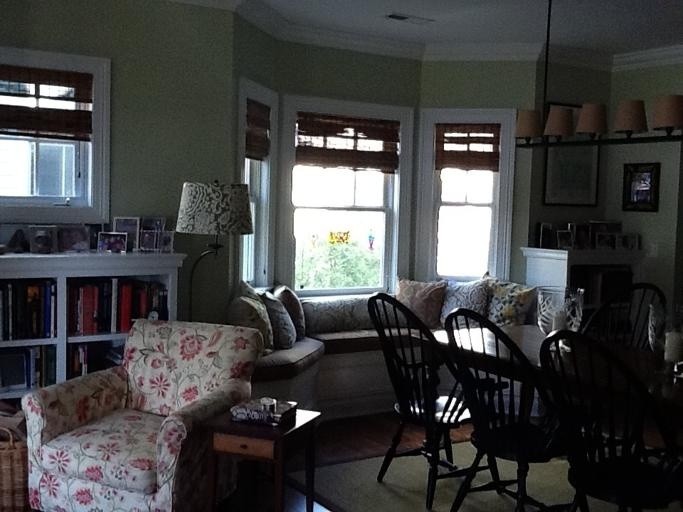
[192,394,325,511]
[409,324,683,511]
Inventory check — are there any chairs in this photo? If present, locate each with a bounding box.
[17,315,267,512]
[365,294,504,511]
[537,332,682,510]
[445,308,591,512]
[575,281,668,461]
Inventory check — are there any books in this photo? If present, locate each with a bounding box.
[0,278,168,391]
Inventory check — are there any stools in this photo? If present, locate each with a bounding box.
[251,336,325,421]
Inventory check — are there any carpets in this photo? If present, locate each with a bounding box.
[280,423,683,512]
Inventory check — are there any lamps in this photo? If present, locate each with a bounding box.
[172,178,254,324]
[511,88,682,158]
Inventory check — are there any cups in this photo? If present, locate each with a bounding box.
[664,328,681,363]
[552,313,567,331]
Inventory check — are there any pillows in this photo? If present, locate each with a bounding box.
[222,275,308,358]
[379,273,541,334]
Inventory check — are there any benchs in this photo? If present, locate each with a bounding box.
[301,292,545,419]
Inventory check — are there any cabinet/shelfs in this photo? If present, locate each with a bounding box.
[0,248,189,408]
[517,241,650,355]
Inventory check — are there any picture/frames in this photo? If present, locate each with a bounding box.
[532,210,646,253]
[0,206,180,263]
[618,161,663,214]
[536,98,604,209]
